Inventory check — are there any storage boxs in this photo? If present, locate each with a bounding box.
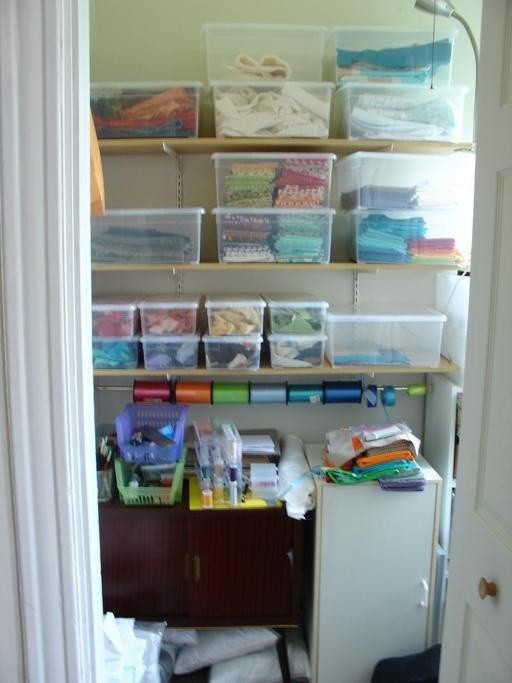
[201,22,324,84]
[332,82,456,144]
[203,79,334,138]
[91,208,207,266]
[334,25,456,88]
[334,150,465,211]
[325,301,448,369]
[261,291,329,336]
[202,330,263,370]
[265,329,327,368]
[139,327,201,369]
[139,293,203,336]
[91,292,148,337]
[210,152,337,208]
[92,331,141,368]
[342,208,465,264]
[204,292,267,337]
[210,206,339,265]
[90,80,203,142]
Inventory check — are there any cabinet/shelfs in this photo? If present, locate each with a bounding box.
[97,506,295,628]
[307,445,448,682]
